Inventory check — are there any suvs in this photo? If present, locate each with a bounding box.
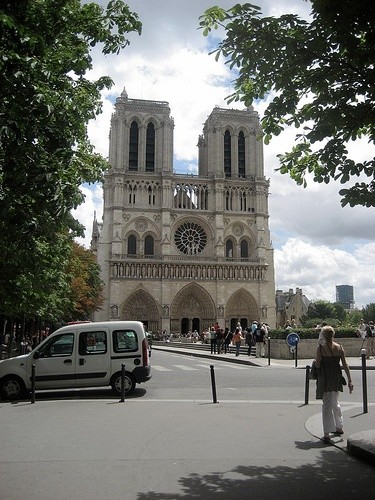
[1,320,151,402]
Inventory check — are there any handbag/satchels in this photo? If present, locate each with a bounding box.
[340,376,347,386]
[309,361,318,380]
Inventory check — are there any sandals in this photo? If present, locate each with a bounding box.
[323,436,331,443]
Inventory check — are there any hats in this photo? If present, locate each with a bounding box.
[253,321,258,324]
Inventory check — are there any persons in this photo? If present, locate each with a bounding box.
[285,319,296,329]
[146,329,180,340]
[317,323,328,344]
[360,319,375,358]
[188,330,199,342]
[315,325,353,440]
[203,320,270,358]
[5,333,46,353]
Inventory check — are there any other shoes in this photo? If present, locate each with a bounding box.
[331,426,342,433]
